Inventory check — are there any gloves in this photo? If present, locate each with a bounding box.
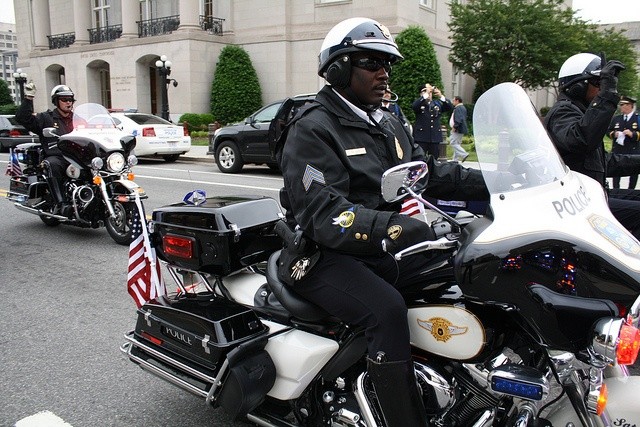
[387,213,436,257]
[600,51,626,92]
[615,131,626,146]
[24,79,37,99]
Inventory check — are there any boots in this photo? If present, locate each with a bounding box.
[366,354,427,426]
[46,176,73,217]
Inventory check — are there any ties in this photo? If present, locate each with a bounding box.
[624,115,627,123]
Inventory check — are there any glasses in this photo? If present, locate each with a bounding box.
[587,78,600,89]
[57,97,73,102]
[354,57,392,72]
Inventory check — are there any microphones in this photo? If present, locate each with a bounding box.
[380,89,400,104]
[67,100,75,108]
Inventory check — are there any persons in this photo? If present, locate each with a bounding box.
[381,89,406,125]
[605,95,640,189]
[540,50,640,243]
[276,13,527,427]
[411,83,452,161]
[14,74,87,214]
[446,96,469,163]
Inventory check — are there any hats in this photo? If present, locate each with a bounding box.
[418,83,434,92]
[618,95,636,106]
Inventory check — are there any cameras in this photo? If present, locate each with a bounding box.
[431,85,437,90]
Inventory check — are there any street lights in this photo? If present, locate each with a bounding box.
[13,68,30,105]
[155,54,178,124]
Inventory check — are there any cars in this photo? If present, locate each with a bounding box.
[212,98,293,173]
[107,107,191,164]
[0,114,40,152]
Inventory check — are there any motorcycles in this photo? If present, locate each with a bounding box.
[4,100,146,246]
[120,82,640,427]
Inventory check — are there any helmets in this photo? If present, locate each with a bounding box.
[51,84,74,105]
[317,17,404,90]
[558,53,603,99]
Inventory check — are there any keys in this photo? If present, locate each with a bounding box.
[290,258,311,280]
